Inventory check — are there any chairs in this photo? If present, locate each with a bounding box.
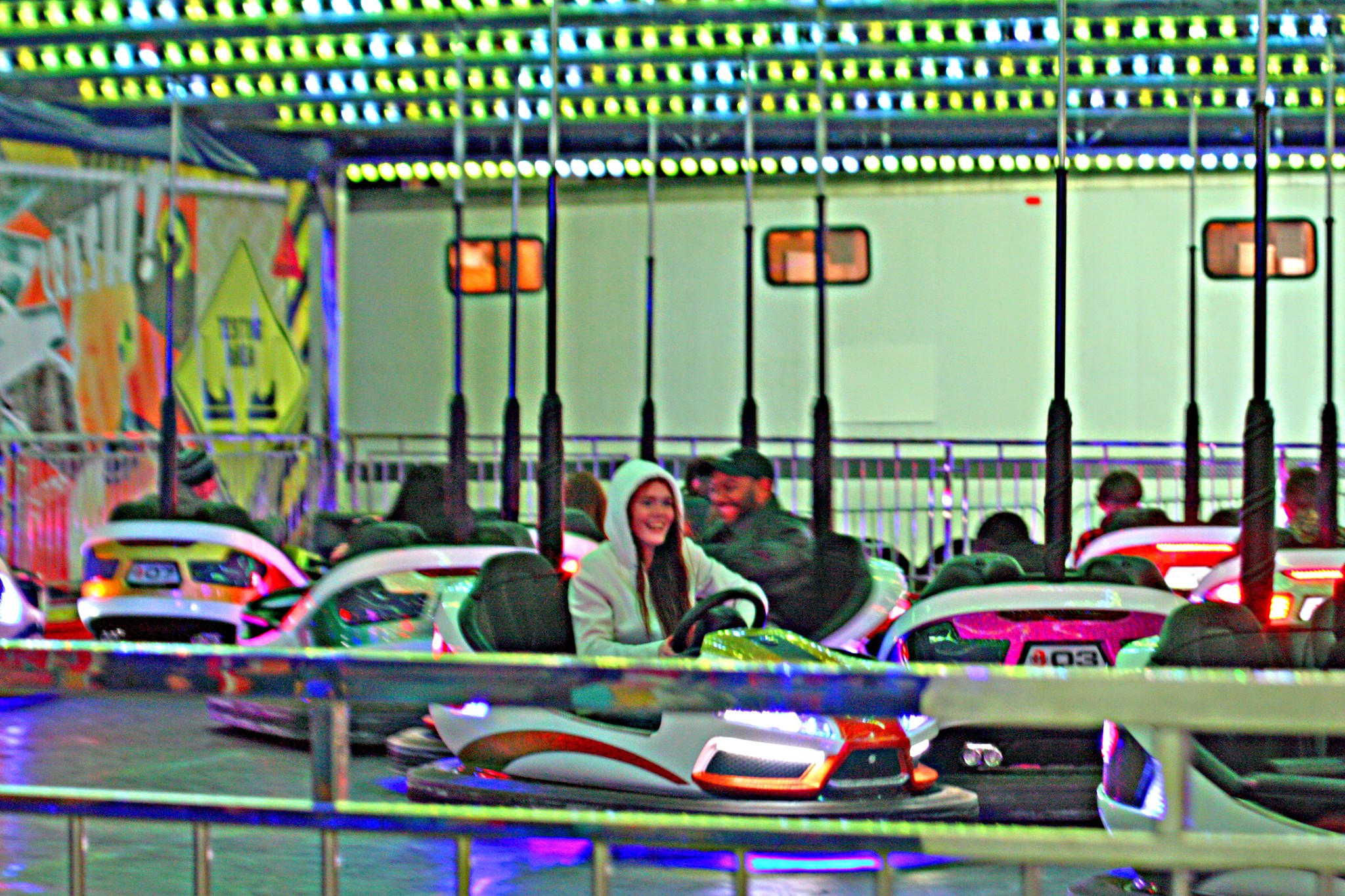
[919,552,1174,598]
[345,518,535,549]
[459,552,580,712]
[1146,600,1345,819]
[798,531,871,644]
[107,499,263,540]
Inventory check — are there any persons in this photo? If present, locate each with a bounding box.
[568,460,770,728]
[1074,471,1143,564]
[976,512,1029,549]
[175,445,217,501]
[681,448,823,631]
[388,465,446,537]
[1276,467,1345,548]
[560,472,606,541]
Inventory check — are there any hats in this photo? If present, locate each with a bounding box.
[694,450,774,484]
